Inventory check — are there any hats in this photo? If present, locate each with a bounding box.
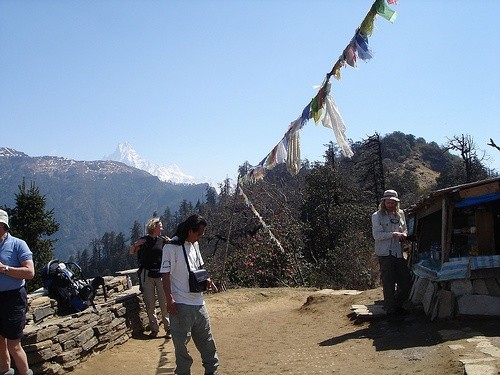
[0,209,10,228]
[381,189,401,202]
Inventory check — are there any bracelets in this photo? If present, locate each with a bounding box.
[4,265,9,274]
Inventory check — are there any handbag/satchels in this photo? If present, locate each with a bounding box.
[180,238,209,292]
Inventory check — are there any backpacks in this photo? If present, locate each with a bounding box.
[43,259,92,313]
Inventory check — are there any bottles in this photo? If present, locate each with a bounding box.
[127,275,132,288]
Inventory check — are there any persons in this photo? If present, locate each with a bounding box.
[0,208,35,375]
[128,217,171,339]
[372,190,411,321]
[160,214,220,375]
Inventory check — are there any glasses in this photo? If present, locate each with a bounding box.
[390,219,400,224]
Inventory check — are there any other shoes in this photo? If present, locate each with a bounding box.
[165,329,172,338]
[149,331,158,338]
[387,309,410,322]
